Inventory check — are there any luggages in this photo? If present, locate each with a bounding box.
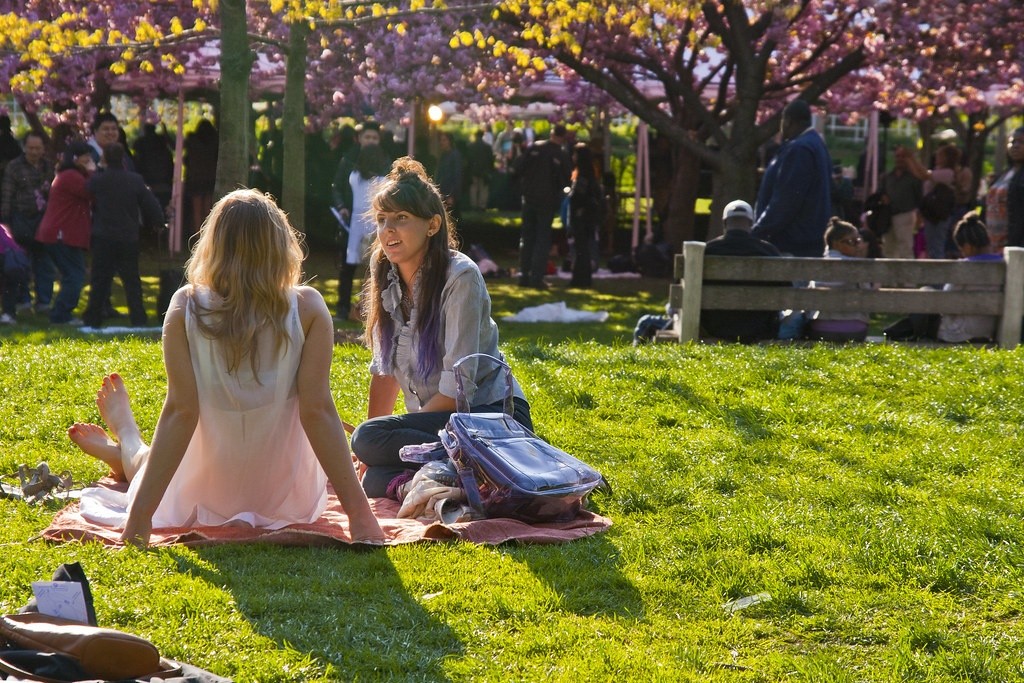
[156,224,188,327]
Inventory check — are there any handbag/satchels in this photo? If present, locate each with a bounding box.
[0,247,31,283]
[867,196,890,236]
[0,610,183,683]
[396,353,602,525]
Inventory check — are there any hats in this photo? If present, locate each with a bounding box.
[722,200,755,223]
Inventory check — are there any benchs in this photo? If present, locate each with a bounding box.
[649,241,1024,348]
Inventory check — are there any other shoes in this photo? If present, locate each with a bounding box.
[521,276,548,289]
[34,303,53,316]
[14,300,32,312]
[0,312,18,324]
[49,318,84,327]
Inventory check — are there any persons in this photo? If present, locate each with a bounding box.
[699,199,780,341]
[351,160,535,501]
[0,96,219,329]
[805,218,869,342]
[829,136,994,288]
[435,116,603,290]
[983,126,1024,255]
[899,211,1006,345]
[248,119,410,323]
[751,97,832,338]
[68,188,386,548]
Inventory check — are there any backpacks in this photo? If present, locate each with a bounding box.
[949,164,975,204]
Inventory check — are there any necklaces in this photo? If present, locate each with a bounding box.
[401,290,414,317]
